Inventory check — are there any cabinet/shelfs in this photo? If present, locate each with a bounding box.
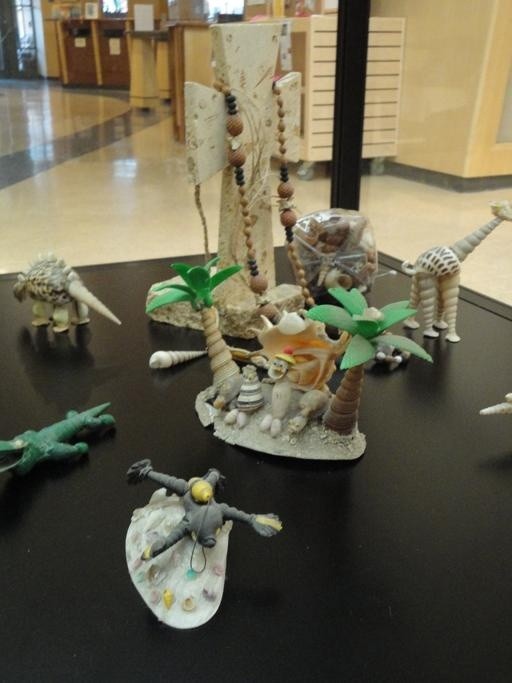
[251,13,410,187]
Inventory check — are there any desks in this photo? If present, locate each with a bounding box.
[2,229,511,682]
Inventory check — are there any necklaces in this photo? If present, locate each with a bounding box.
[211,79,329,324]
